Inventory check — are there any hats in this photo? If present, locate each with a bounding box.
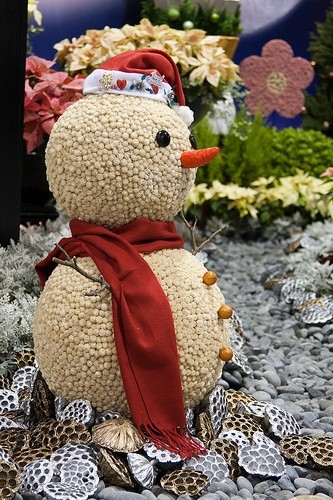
[82,48,195,126]
